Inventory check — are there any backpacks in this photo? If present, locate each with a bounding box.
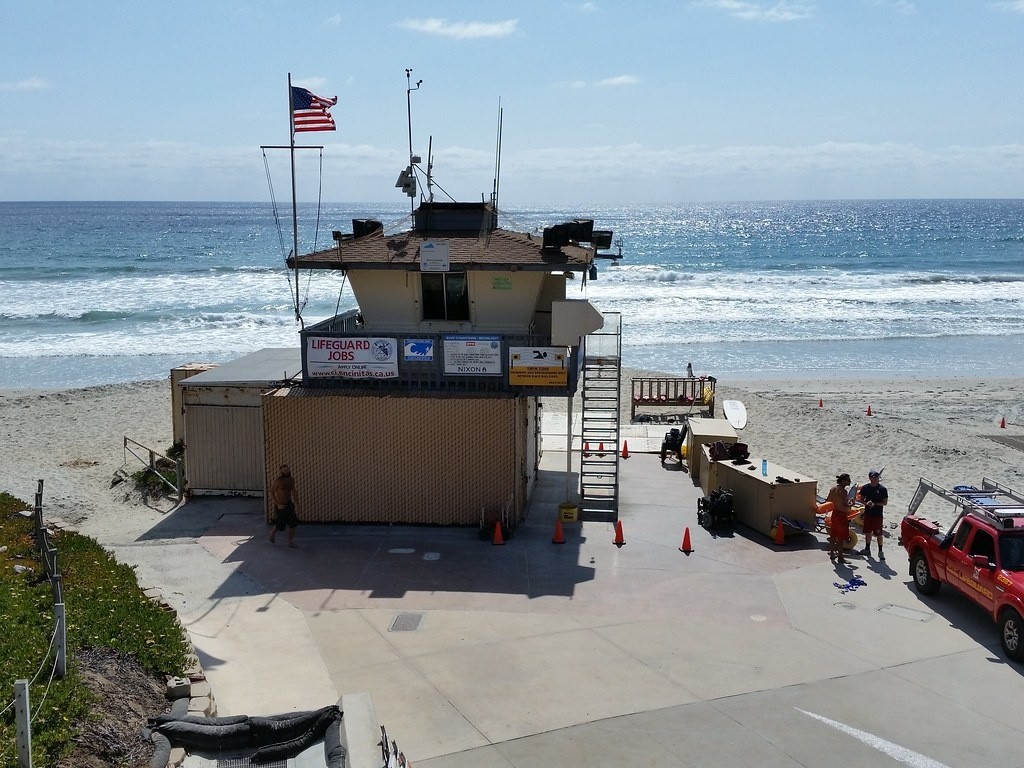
[710,440,730,461]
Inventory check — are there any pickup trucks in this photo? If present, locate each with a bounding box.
[899,472,1023,663]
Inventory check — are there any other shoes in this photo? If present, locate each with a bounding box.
[269,534,275,544]
[859,549,871,555]
[288,543,297,548]
[878,552,886,560]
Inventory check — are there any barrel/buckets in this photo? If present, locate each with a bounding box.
[670,429,679,438]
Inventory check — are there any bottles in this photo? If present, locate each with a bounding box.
[762,460,767,476]
[687,363,692,377]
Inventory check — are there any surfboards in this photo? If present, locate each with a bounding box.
[723,400,747,429]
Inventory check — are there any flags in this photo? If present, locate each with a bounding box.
[290,86,338,133]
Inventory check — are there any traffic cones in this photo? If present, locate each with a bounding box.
[492,522,505,545]
[620,440,631,458]
[819,399,823,407]
[677,526,694,553]
[773,520,787,546]
[865,405,873,416]
[583,442,592,456]
[611,521,626,544]
[1001,416,1007,428]
[596,441,606,456]
[551,518,566,544]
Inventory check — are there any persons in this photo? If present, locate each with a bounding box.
[826,473,855,564]
[269,465,303,549]
[858,472,888,560]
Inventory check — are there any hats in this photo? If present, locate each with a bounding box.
[869,471,880,478]
